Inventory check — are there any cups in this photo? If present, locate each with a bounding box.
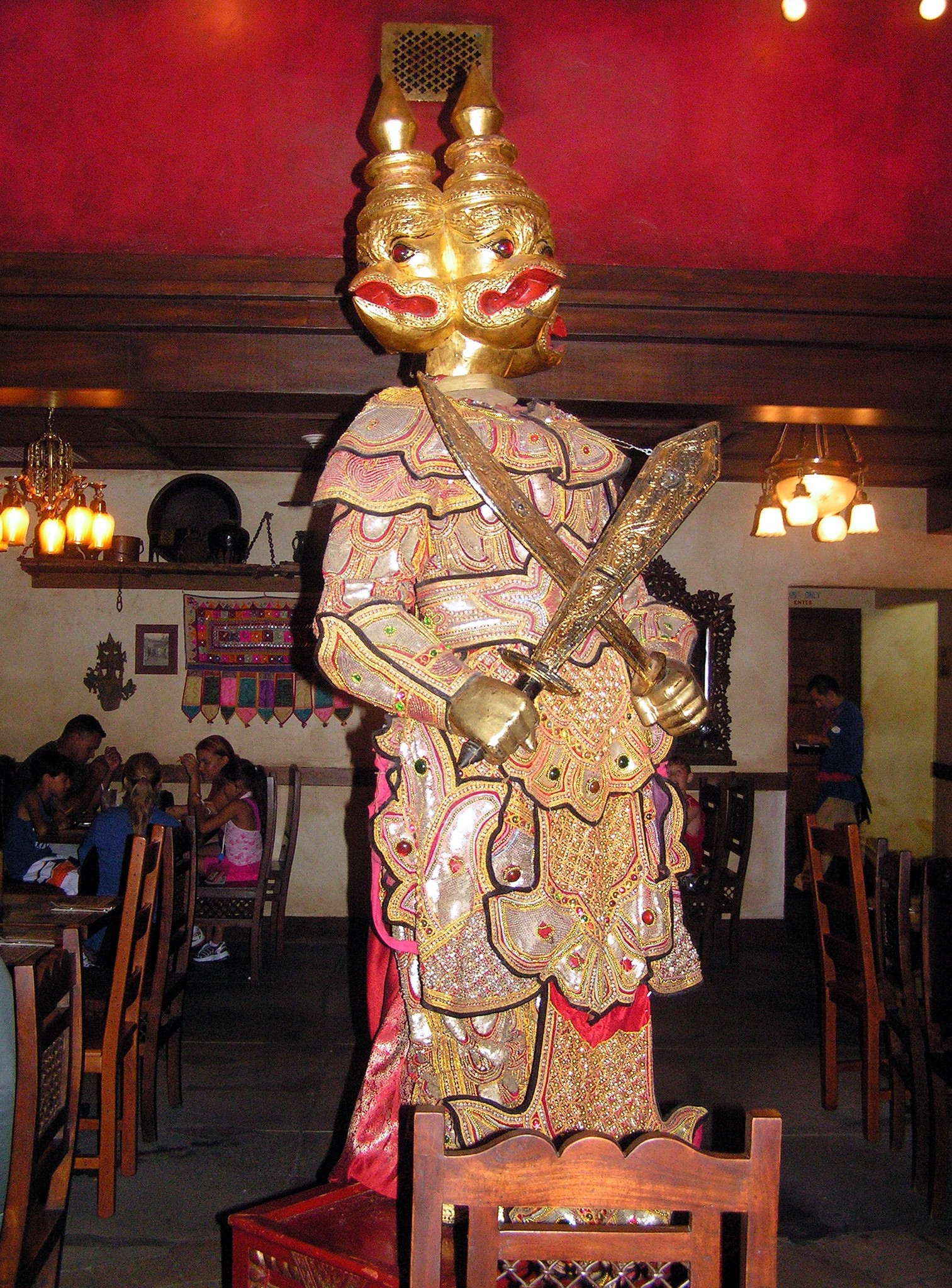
[100,789,117,812]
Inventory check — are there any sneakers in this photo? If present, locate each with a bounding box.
[191,940,230,962]
[191,925,204,948]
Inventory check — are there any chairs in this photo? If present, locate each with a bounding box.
[685,774,734,966]
[85,809,208,1143]
[73,821,173,1220]
[408,1100,786,1288]
[261,763,307,957]
[804,813,952,1226]
[194,765,283,982]
[715,780,764,960]
[0,921,93,1288]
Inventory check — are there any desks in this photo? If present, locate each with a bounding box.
[0,891,125,975]
[33,819,218,852]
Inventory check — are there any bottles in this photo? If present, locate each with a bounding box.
[208,518,250,564]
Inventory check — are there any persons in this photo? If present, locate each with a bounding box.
[312,63,709,1288]
[666,758,706,879]
[4,714,268,968]
[795,673,865,893]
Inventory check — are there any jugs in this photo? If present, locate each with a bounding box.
[292,530,308,562]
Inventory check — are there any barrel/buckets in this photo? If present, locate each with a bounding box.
[103,534,144,562]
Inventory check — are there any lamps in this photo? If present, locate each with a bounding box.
[752,425,881,543]
[0,408,118,558]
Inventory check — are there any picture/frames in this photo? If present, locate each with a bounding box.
[134,623,179,676]
